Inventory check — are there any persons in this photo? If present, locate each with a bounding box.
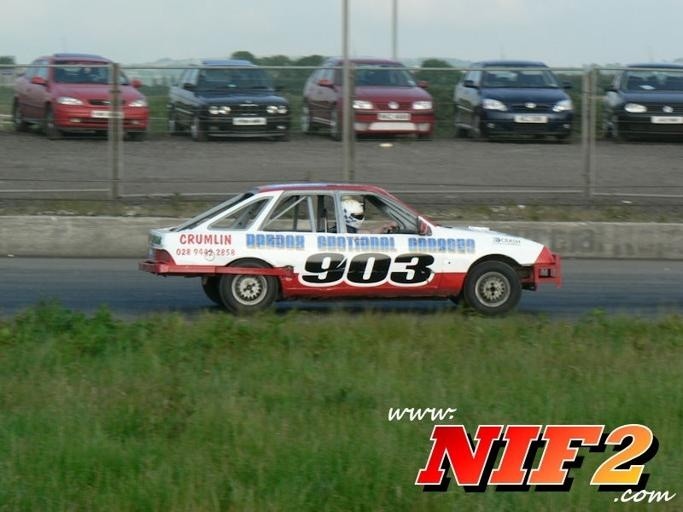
[327,200,365,233]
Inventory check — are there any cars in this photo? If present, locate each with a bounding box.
[165,58,291,139]
[299,56,435,140]
[601,64,682,142]
[12,51,149,139]
[138,182,561,314]
[454,60,573,142]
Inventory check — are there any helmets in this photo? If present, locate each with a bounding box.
[342,200,364,229]
